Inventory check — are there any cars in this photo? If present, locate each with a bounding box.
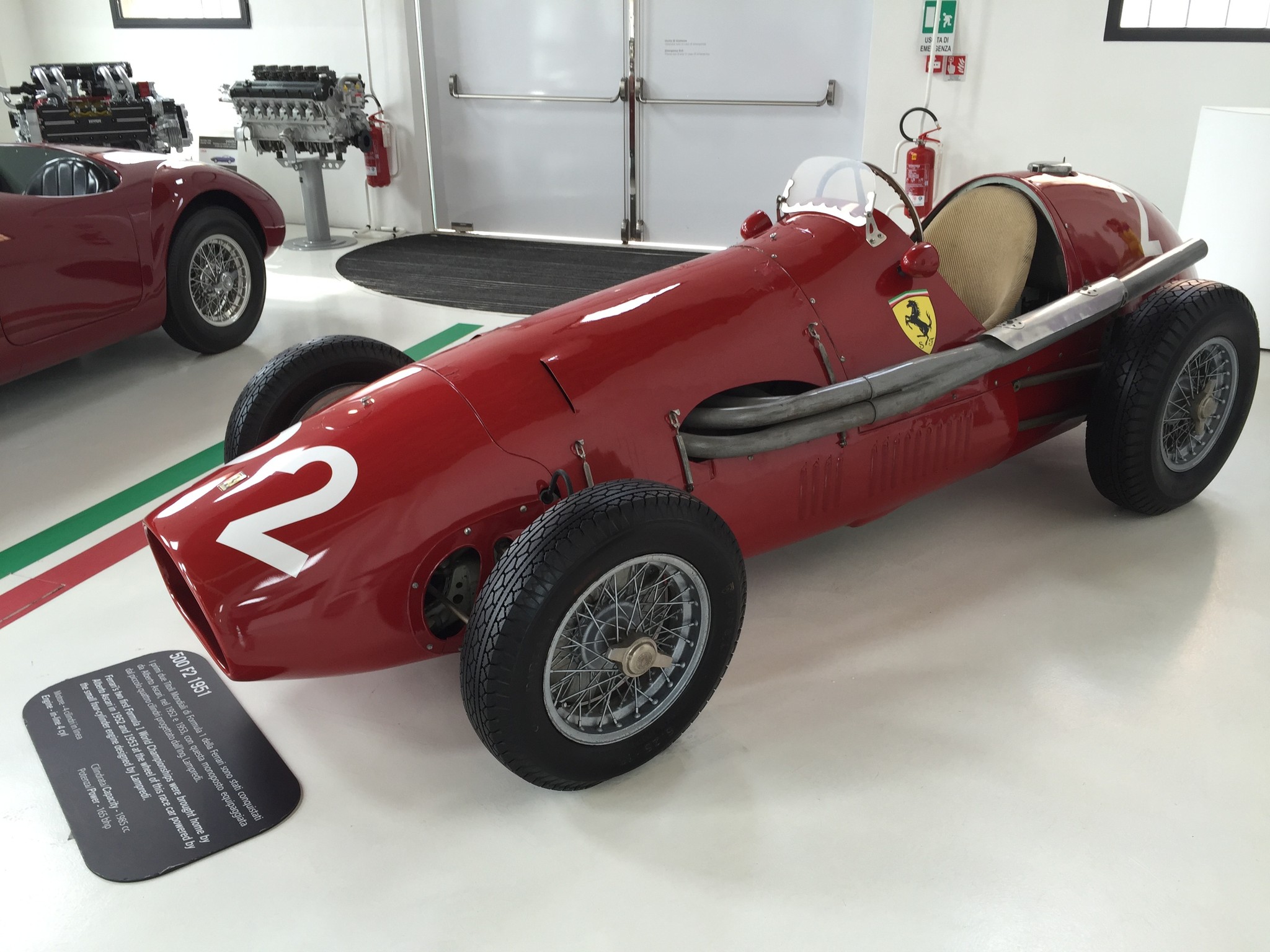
[141,151,1262,796]
[0,140,288,386]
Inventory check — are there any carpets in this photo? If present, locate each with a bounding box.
[336,232,711,315]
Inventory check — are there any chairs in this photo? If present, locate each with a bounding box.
[914,185,1039,331]
[21,156,112,197]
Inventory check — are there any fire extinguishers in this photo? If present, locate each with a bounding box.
[900,107,942,219]
[361,95,392,188]
